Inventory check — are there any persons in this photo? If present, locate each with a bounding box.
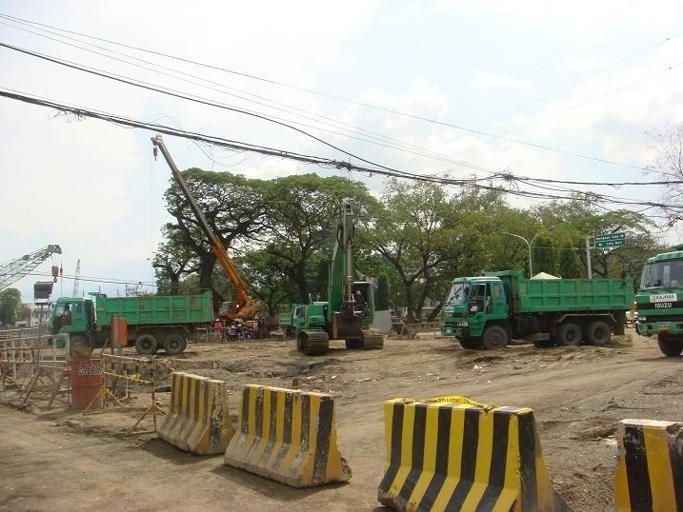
[354,289,366,318]
[60,305,71,325]
[212,318,238,337]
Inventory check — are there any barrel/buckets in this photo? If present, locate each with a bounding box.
[67,357,105,410]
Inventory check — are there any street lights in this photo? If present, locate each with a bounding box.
[501,226,558,278]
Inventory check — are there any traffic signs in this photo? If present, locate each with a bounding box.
[593,233,626,248]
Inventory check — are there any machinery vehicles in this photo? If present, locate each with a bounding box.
[297,196,383,353]
[150,133,266,341]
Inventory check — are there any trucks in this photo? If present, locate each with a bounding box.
[437,269,633,349]
[633,242,682,356]
[46,290,215,356]
[276,303,303,336]
